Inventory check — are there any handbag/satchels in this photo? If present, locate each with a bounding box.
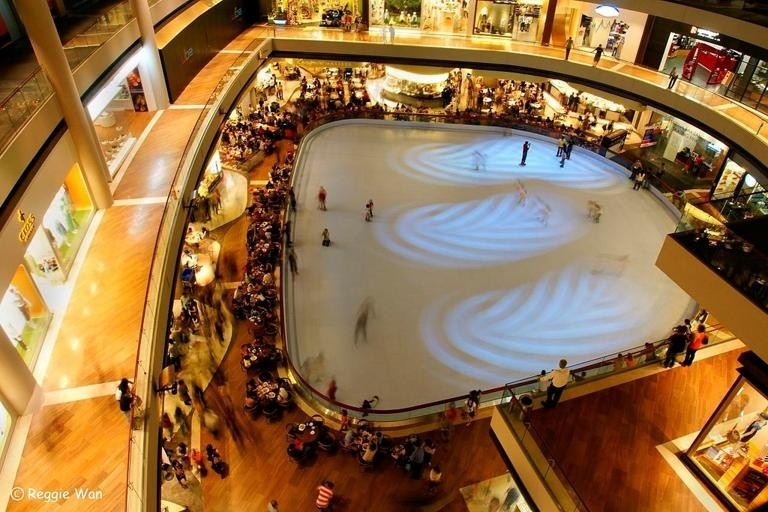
[702,332,709,345]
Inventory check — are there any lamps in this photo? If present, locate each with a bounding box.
[594,1,620,17]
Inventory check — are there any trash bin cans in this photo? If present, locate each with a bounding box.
[515,394,535,425]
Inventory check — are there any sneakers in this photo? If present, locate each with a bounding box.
[541,399,555,408]
[661,360,693,367]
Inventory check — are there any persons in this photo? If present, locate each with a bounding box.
[167,1,767,512]
[116,377,136,424]
